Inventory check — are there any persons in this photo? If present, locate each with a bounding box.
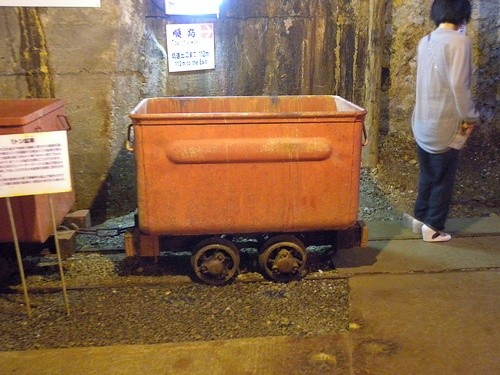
[411,0,481,241]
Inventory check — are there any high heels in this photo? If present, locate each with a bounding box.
[413,219,451,241]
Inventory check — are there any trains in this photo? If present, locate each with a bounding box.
[0,96,368,286]
